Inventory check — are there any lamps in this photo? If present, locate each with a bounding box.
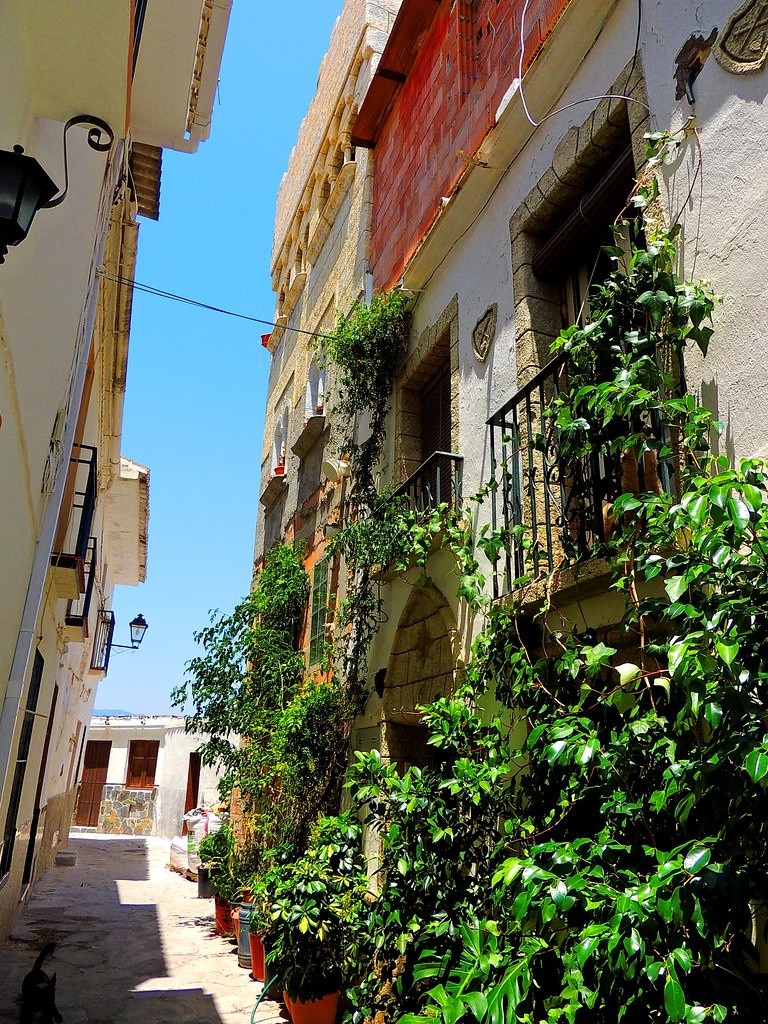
[0,144,61,265]
[105,612,148,649]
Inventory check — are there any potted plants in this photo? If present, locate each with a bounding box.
[274,447,286,474]
[196,807,369,1024]
